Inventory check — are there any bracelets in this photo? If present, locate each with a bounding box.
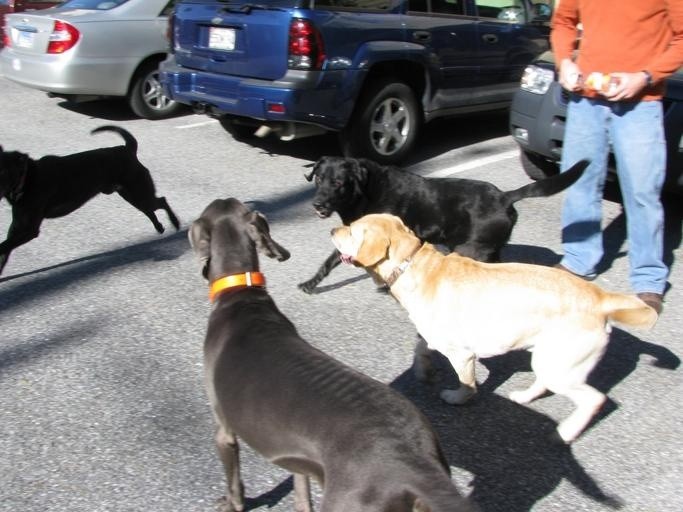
[642,70,652,88]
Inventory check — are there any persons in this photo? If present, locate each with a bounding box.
[547,0,682,315]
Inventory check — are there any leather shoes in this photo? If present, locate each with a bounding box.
[634,289,665,314]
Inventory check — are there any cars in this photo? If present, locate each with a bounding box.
[512,47,683,198]
[1,0,182,121]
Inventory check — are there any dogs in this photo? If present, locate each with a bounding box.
[187,198,483,512]
[329,213,658,444]
[0,125,180,276]
[296,155,593,295]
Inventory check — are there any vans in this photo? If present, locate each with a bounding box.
[159,1,553,165]
